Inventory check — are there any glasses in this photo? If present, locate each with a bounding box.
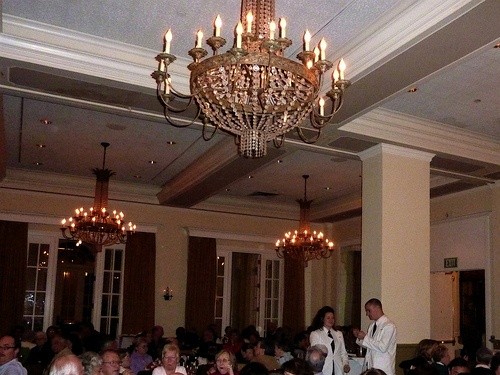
[216,359,227,364]
[0,345,16,350]
[104,360,123,367]
[163,356,177,362]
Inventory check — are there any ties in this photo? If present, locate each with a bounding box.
[328,330,335,354]
[372,322,376,336]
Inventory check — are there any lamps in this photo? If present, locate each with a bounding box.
[163,286,173,300]
[151,0,353,160]
[275,174,335,267]
[61,142,136,252]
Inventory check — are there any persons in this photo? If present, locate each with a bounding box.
[353,298,397,375]
[399,339,500,375]
[309,306,350,375]
[0,321,325,375]
[360,368,386,375]
